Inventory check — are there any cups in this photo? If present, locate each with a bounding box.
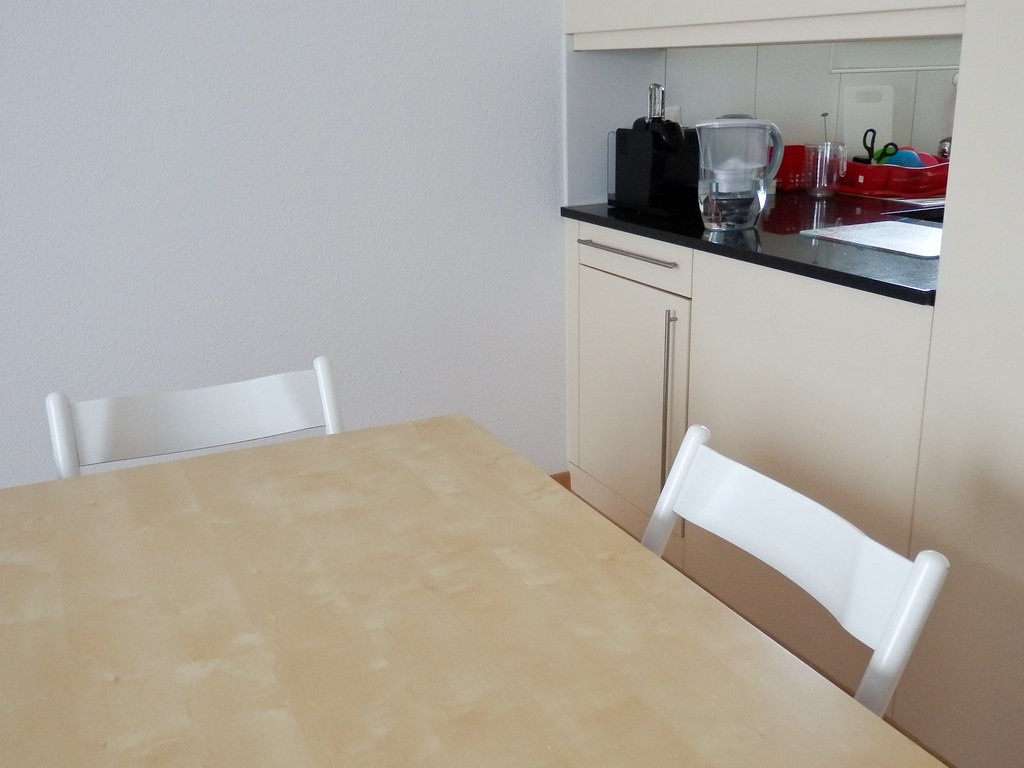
[804,143,847,192]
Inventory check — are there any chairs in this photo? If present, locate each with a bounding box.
[46,357,340,476]
[640,425,951,719]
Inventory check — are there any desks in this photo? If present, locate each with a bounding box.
[1,414,942,768]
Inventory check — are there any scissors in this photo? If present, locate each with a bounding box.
[863,129,898,164]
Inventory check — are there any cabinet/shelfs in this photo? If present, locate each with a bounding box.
[563,0,1024,768]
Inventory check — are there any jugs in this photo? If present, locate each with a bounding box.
[694,113,783,232]
